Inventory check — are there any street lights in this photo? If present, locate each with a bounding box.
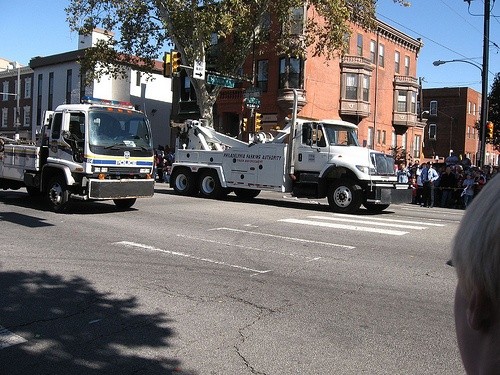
[432,59,487,170]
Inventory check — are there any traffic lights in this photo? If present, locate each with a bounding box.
[171,50,182,74]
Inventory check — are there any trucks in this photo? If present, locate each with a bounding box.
[0,96,156,213]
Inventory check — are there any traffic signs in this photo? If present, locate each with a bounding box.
[193,60,206,80]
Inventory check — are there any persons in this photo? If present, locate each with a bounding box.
[445,173,500,375]
[154,145,175,184]
[393,152,500,209]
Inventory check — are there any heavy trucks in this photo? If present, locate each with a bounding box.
[170,86,399,215]
